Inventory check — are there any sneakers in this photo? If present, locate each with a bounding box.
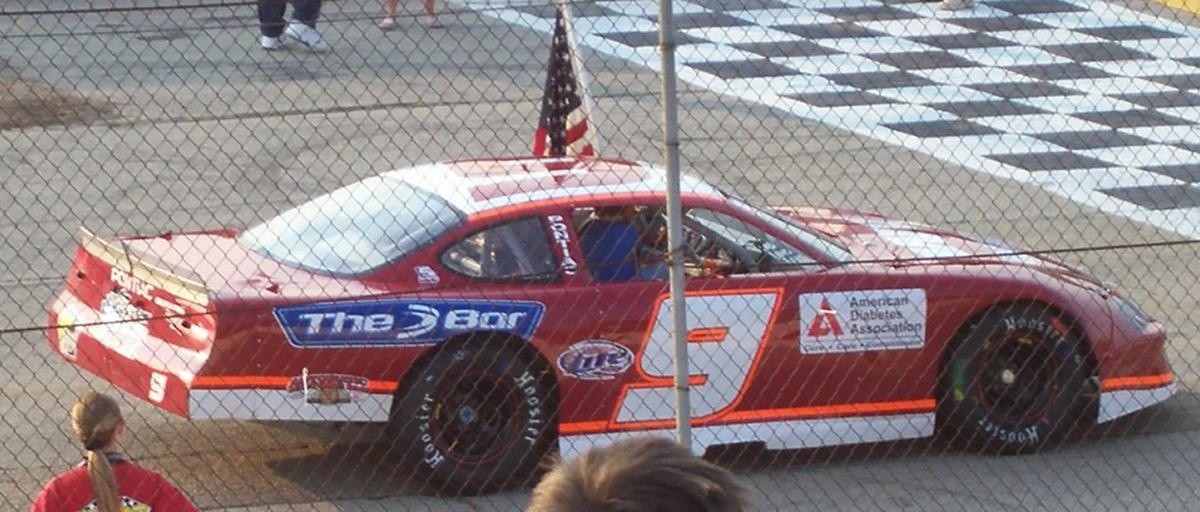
[258,21,329,54]
[378,17,398,30]
[424,15,442,29]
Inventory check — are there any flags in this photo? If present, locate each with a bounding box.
[534,0,601,159]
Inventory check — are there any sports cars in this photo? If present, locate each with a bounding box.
[39,156,1181,500]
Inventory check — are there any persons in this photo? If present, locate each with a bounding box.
[379,1,441,29]
[254,1,331,52]
[524,431,756,511]
[28,390,201,511]
[579,201,682,285]
